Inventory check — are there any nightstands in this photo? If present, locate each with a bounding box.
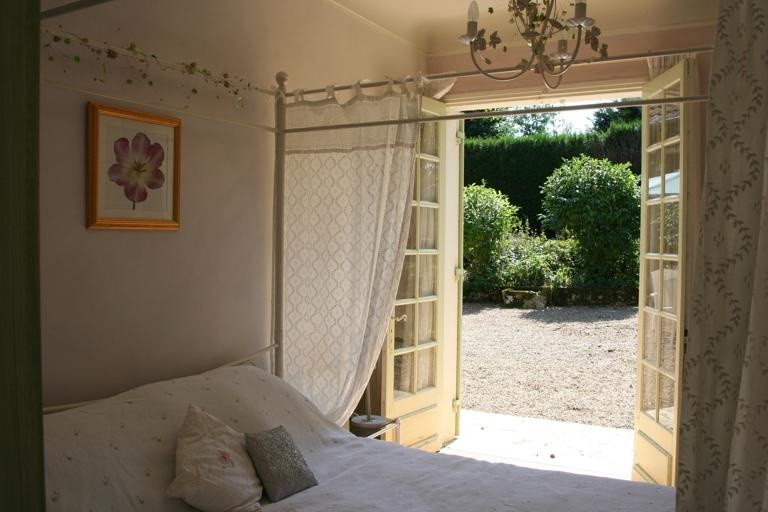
[345,416,403,444]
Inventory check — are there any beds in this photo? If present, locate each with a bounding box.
[41,363,682,511]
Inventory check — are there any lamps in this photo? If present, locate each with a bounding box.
[453,0,611,90]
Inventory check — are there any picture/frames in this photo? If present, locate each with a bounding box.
[83,98,183,233]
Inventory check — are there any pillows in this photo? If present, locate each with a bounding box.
[240,424,320,502]
[168,399,267,510]
